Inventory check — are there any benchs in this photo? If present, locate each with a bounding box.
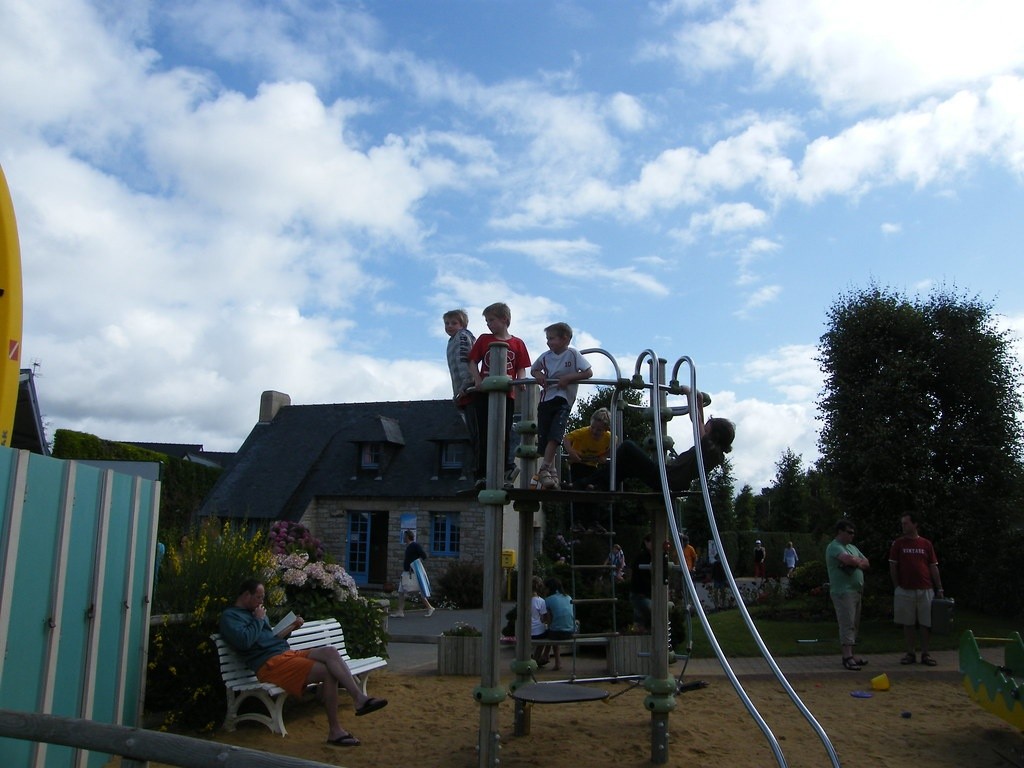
[210,618,386,735]
[528,636,610,644]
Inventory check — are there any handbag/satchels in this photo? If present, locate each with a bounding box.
[930,596,954,635]
[401,571,419,592]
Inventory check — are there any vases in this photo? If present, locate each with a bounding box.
[437,636,483,677]
[610,636,648,676]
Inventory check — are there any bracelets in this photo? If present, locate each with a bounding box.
[937,589,944,592]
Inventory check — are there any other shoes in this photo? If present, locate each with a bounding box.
[539,472,555,488]
[548,475,560,490]
[553,664,562,669]
[424,607,435,617]
[390,613,406,619]
[538,656,550,665]
[503,463,520,486]
[474,477,488,488]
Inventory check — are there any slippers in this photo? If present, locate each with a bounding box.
[327,731,362,747]
[355,696,388,716]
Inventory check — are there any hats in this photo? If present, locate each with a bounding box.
[756,540,762,545]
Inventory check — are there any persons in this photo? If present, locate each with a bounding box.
[530,322,593,488]
[783,542,798,579]
[220,578,388,747]
[444,303,531,489]
[752,540,766,586]
[889,513,946,666]
[389,530,435,617]
[562,408,618,535]
[543,575,574,670]
[826,520,870,670]
[603,534,730,608]
[569,385,736,505]
[530,576,550,665]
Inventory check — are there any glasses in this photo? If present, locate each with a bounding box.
[839,530,856,535]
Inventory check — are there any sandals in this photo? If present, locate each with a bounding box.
[843,656,860,670]
[921,653,936,666]
[901,654,917,664]
[852,652,868,665]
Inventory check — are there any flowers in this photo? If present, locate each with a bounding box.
[623,621,651,636]
[441,618,480,635]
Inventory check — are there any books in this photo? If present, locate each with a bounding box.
[273,611,299,639]
